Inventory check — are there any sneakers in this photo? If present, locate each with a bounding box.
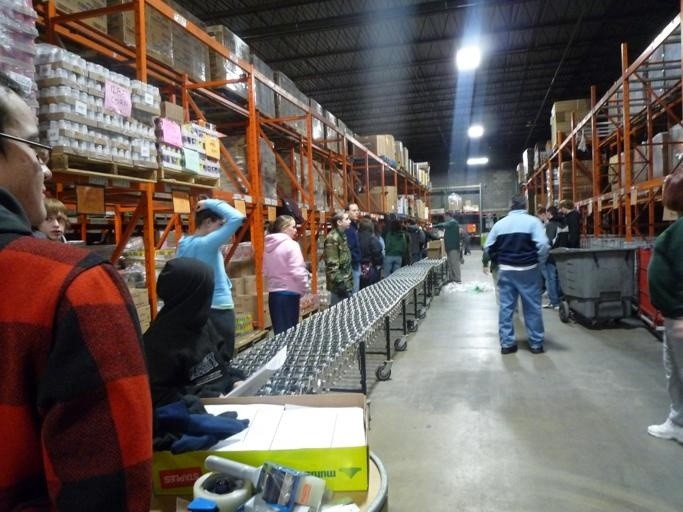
[531,346,543,353]
[502,346,513,353]
[648,418,683,445]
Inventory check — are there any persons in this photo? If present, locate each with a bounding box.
[324,202,468,308]
[482,215,520,313]
[141,258,245,407]
[177,199,245,361]
[1,82,153,511]
[262,215,311,336]
[40,199,74,244]
[536,202,580,308]
[485,195,550,353]
[647,173,683,444]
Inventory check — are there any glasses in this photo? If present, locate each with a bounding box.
[0,133,54,165]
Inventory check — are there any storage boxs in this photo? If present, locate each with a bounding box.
[424,239,446,259]
[149,392,368,497]
[311,97,430,190]
[312,111,430,318]
[516,163,523,184]
[35,0,309,135]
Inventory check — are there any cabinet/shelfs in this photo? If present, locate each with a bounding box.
[142,0,256,198]
[250,65,312,207]
[520,87,595,239]
[262,203,315,340]
[35,166,152,353]
[623,13,682,188]
[35,0,145,180]
[153,183,262,352]
[594,192,628,253]
[593,47,628,190]
[626,183,683,343]
[431,183,481,238]
[481,208,511,233]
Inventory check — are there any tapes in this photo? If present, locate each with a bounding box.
[193,472,252,512]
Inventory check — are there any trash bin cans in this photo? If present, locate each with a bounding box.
[549,247,634,330]
